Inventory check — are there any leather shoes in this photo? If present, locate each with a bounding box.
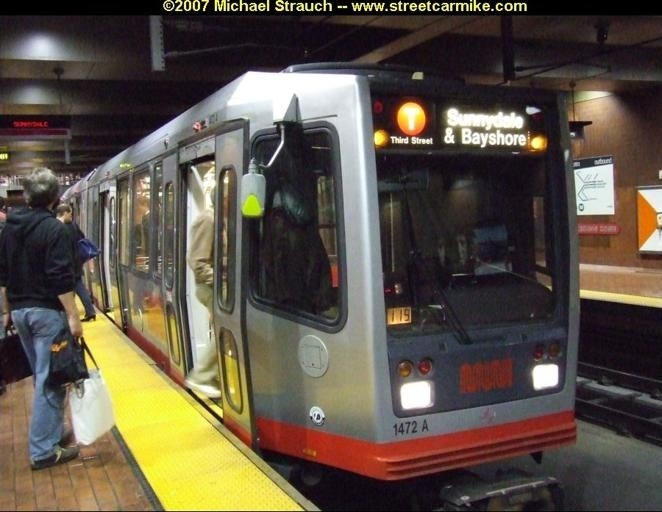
[66,367,116,446]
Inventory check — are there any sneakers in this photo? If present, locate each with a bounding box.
[183,377,221,398]
[30,428,80,471]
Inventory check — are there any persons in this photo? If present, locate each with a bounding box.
[0,190,102,394]
[0,164,83,473]
[446,227,479,271]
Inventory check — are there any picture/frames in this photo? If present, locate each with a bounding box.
[633,183,662,257]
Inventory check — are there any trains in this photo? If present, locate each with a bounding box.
[59,60,582,511]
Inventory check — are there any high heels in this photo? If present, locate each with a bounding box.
[80,314,96,322]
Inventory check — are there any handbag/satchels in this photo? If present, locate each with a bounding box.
[0,326,34,384]
[72,221,102,261]
[48,328,90,388]
